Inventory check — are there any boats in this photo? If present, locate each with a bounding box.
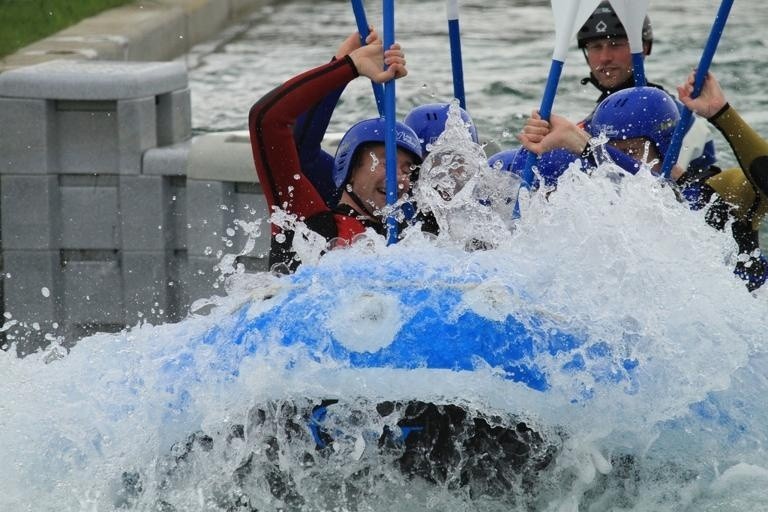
[38,243,768,511]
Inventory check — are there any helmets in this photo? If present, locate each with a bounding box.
[331,117,423,189]
[487,86,680,190]
[403,102,478,155]
[577,1,651,54]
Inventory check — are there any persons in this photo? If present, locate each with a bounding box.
[247,1,767,297]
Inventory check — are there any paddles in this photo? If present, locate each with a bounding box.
[524,1,654,188]
